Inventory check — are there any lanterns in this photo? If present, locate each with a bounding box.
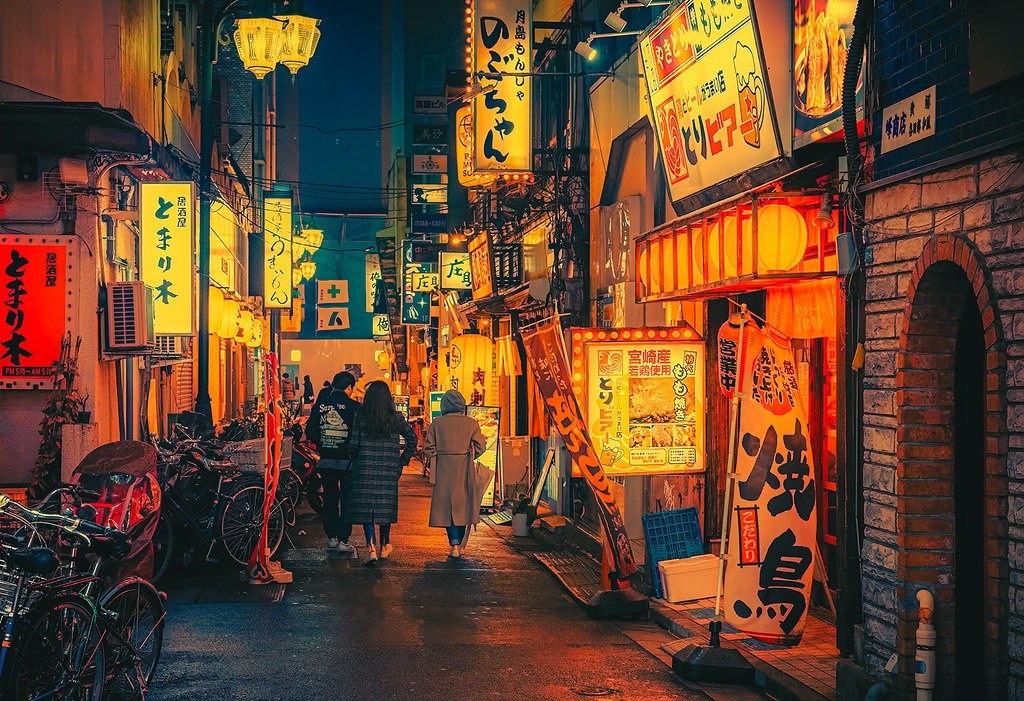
[448,329,493,407]
[716,312,763,400]
[639,203,809,297]
[377,351,391,371]
[420,366,431,389]
[196,284,264,347]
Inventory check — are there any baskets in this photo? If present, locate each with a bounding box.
[0,543,59,613]
[156,461,170,483]
[224,436,294,473]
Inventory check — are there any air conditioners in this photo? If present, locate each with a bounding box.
[105,278,157,353]
[153,335,187,361]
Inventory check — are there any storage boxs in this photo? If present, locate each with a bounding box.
[656,553,726,603]
[0,486,29,530]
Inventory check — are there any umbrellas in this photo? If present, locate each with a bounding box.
[472,462,495,532]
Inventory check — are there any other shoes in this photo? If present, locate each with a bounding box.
[448,546,465,558]
[327,538,338,550]
[379,544,393,558]
[363,551,378,566]
[337,541,356,552]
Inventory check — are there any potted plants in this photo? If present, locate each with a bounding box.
[71,387,92,424]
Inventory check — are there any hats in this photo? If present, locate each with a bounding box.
[440,389,466,415]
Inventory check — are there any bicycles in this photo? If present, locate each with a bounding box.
[0,398,340,701]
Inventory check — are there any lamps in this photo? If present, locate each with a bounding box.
[603,0,673,34]
[573,29,644,61]
[636,0,653,9]
[810,191,836,230]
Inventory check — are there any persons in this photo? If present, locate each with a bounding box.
[301,375,314,413]
[422,389,486,559]
[316,372,340,404]
[282,372,296,407]
[304,371,363,554]
[344,380,418,568]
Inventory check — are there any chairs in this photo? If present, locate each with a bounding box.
[55,438,164,561]
